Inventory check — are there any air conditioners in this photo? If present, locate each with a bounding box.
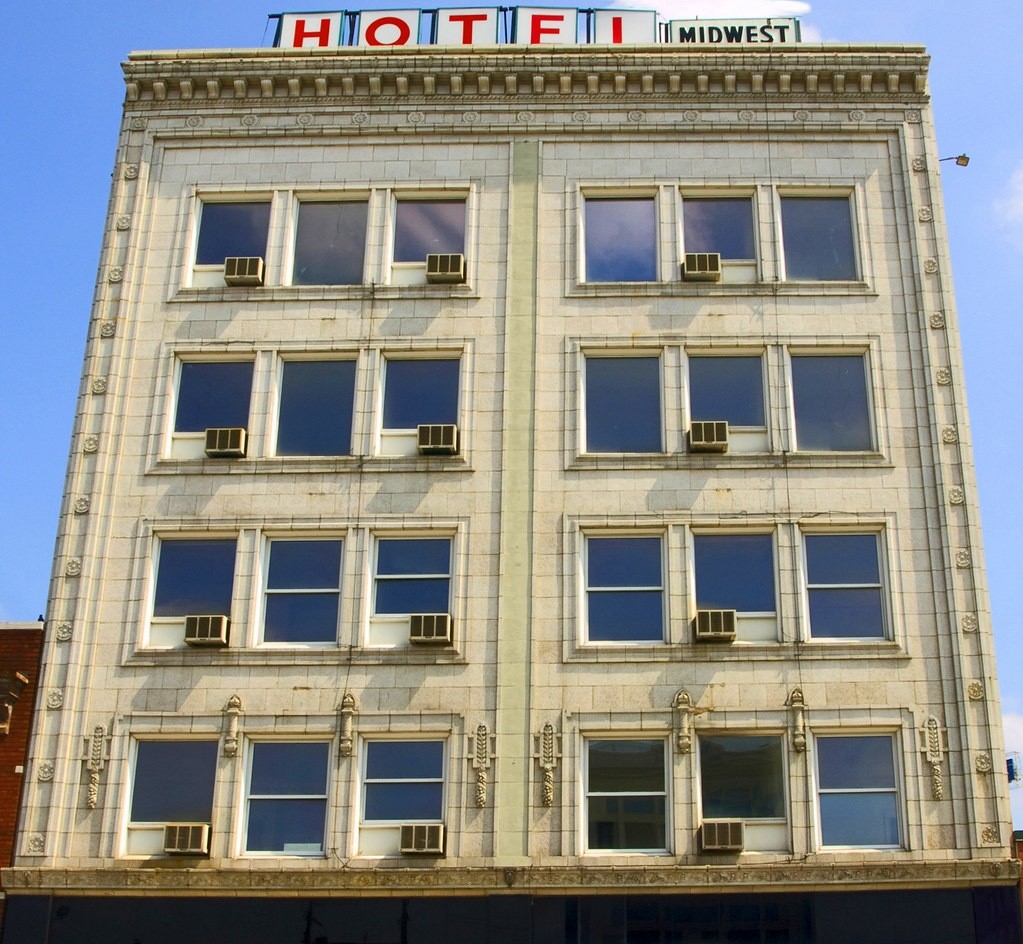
[689,421,729,451]
[685,252,722,281]
[417,424,457,455]
[203,427,247,458]
[399,824,446,854]
[703,819,745,850]
[407,614,452,646]
[182,614,230,648]
[426,253,464,284]
[696,609,738,642]
[163,825,211,857]
[223,257,264,286]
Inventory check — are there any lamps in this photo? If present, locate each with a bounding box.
[939,153,970,166]
